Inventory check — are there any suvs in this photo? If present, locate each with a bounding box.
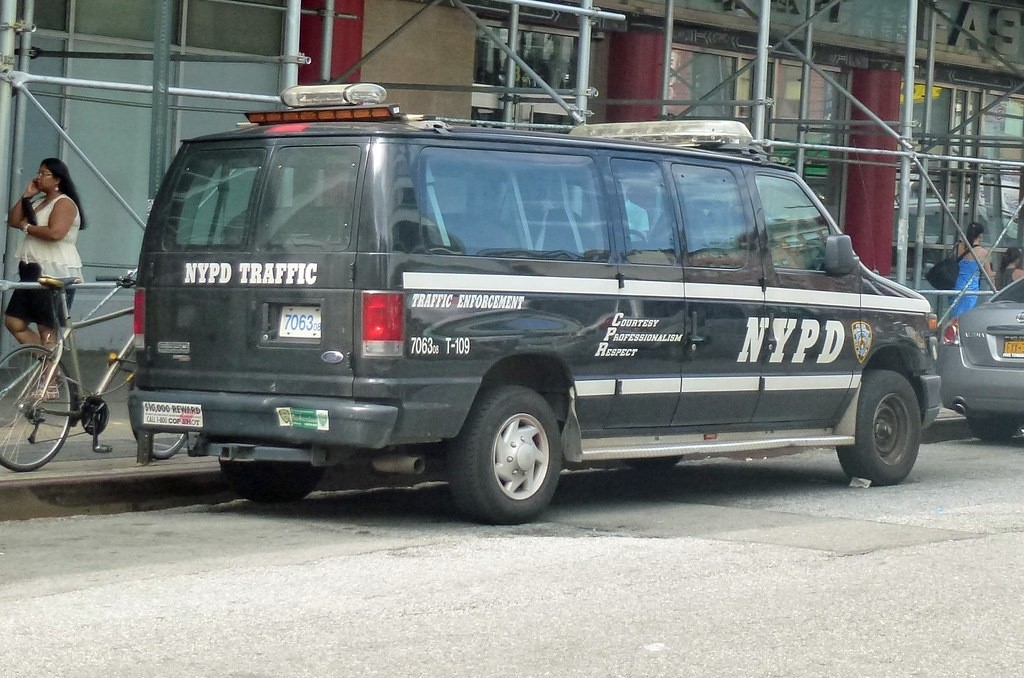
[128,82,942,526]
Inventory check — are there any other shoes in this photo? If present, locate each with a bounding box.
[46,371,68,388]
[32,389,59,400]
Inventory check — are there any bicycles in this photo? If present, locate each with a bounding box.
[0,268,189,472]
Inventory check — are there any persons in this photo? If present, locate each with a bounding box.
[626,181,656,242]
[996,247,1024,291]
[4,158,87,399]
[949,223,995,319]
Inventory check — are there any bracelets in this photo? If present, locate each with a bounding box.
[23,224,31,235]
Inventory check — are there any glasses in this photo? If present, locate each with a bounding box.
[35,171,53,177]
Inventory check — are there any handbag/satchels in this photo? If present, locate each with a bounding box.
[924,259,959,291]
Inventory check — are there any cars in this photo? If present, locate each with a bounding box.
[937,275,1024,423]
